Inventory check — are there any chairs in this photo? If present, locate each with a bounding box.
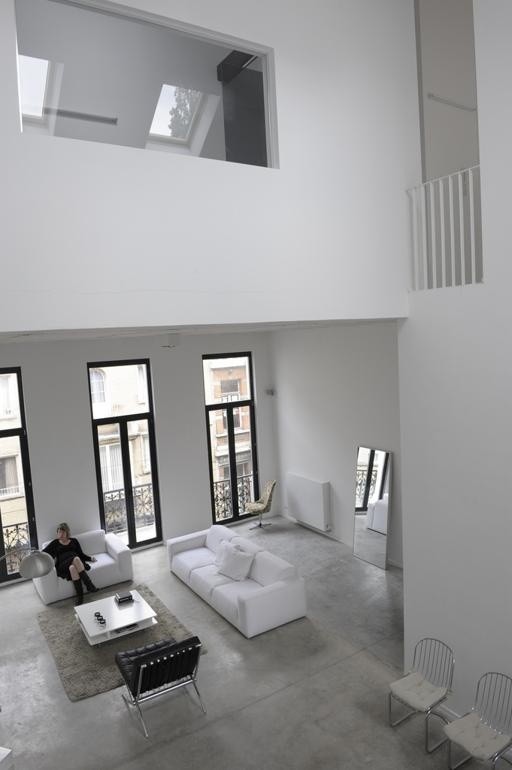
[387,637,512,770]
[32,529,134,608]
[244,478,278,531]
[114,635,208,739]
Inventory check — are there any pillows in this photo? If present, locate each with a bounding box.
[213,538,257,582]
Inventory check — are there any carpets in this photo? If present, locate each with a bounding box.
[33,581,209,705]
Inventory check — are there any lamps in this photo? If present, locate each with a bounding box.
[0,546,57,581]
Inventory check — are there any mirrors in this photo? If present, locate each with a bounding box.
[351,444,394,579]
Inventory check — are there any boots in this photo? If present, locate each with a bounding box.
[79,571,99,592]
[73,578,83,605]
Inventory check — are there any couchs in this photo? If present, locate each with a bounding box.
[165,523,308,639]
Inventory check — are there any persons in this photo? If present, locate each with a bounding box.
[41,523,99,606]
